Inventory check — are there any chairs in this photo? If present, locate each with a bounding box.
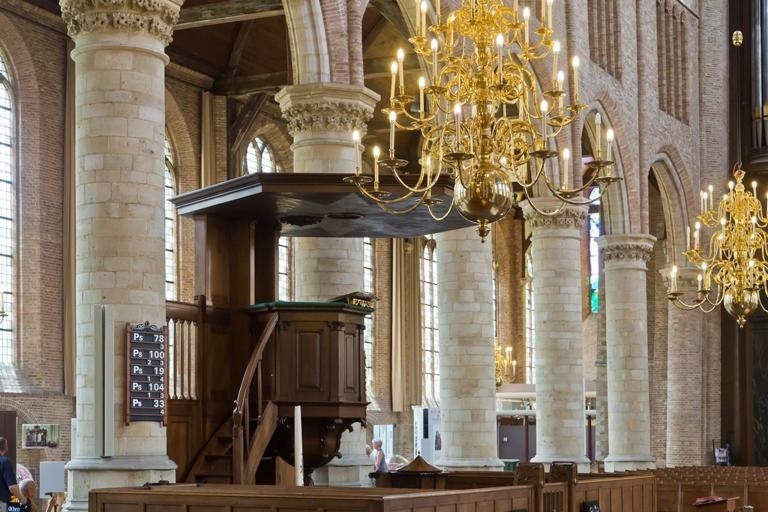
[513,459,545,486]
[603,463,768,511]
[548,459,578,482]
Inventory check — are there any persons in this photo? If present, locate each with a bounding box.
[372,439,388,472]
[366,445,376,470]
[20,479,56,512]
[0,436,27,512]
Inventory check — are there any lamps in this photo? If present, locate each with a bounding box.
[339,0,626,245]
[666,1,768,332]
[489,219,519,392]
